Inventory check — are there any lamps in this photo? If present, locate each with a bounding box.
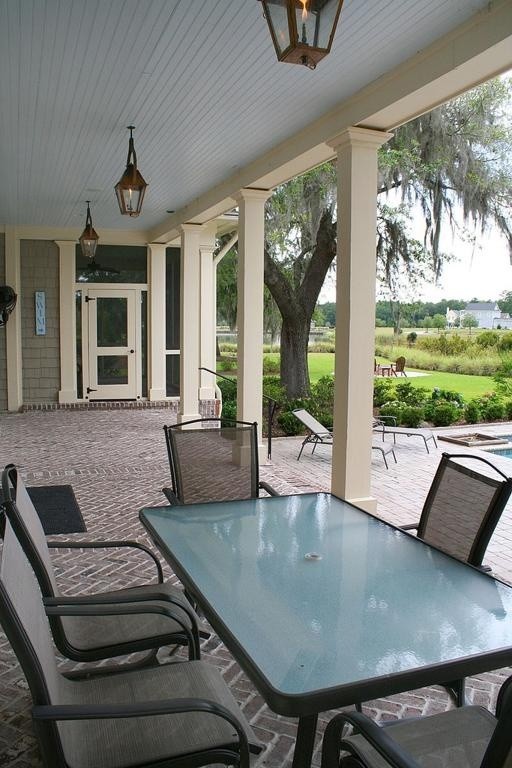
[113,126,149,218]
[259,0,344,68]
[79,201,100,259]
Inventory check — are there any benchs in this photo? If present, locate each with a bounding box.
[293,406,437,470]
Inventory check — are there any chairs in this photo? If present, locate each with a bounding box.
[1,463,212,684]
[0,507,266,767]
[321,677,512,768]
[397,452,511,572]
[293,406,437,470]
[390,357,407,378]
[162,418,280,507]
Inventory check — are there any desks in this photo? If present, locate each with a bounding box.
[138,491,512,767]
[381,367,390,377]
